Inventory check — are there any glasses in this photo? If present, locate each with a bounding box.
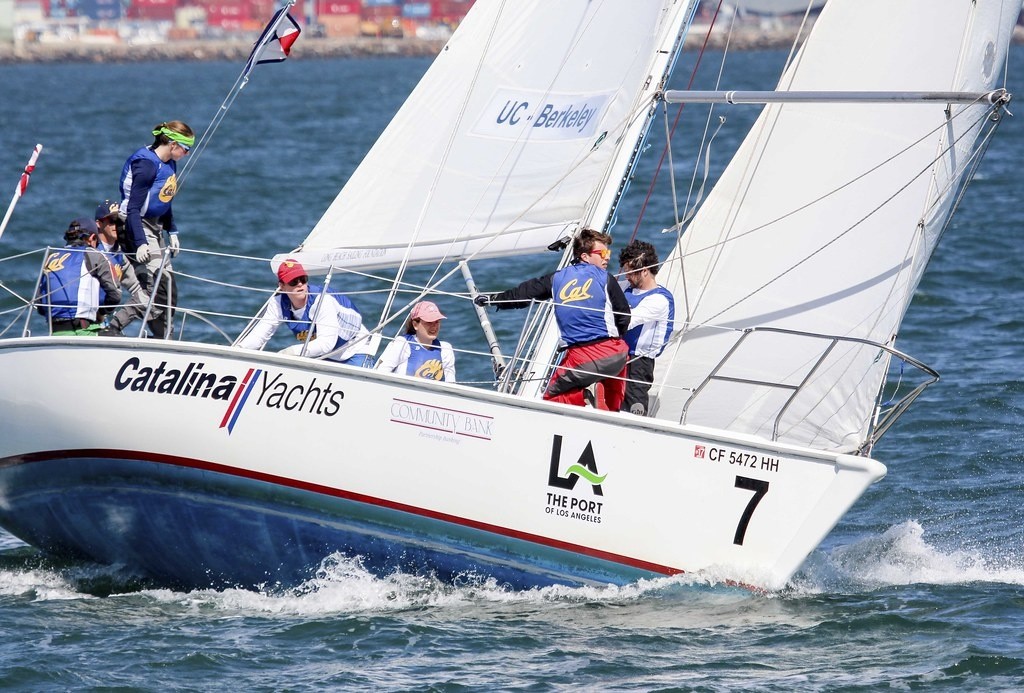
[586,249,611,258]
[100,218,118,224]
[169,140,191,152]
[288,275,308,286]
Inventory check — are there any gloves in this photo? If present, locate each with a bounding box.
[136,244,151,264]
[169,234,180,258]
[474,295,488,307]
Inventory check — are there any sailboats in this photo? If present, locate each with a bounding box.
[0,1,1024,602]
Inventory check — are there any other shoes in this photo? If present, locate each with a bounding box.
[99,325,125,337]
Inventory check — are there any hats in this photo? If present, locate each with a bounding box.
[68,217,98,234]
[411,301,448,322]
[278,259,308,284]
[95,199,122,219]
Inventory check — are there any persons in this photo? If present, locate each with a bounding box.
[475,229,631,409]
[39,218,120,336]
[100,121,195,339]
[619,241,675,415]
[375,301,457,384]
[239,260,375,367]
[94,201,145,306]
